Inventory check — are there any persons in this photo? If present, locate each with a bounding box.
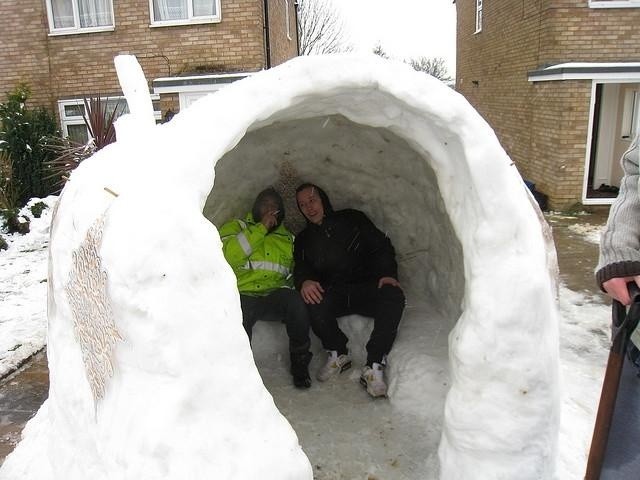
[594,113,640,480]
[294,182,406,398]
[217,189,313,388]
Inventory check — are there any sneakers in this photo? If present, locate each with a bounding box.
[360,362,387,397]
[290,365,312,388]
[317,350,352,381]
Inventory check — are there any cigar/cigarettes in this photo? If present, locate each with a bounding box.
[271,210,281,215]
